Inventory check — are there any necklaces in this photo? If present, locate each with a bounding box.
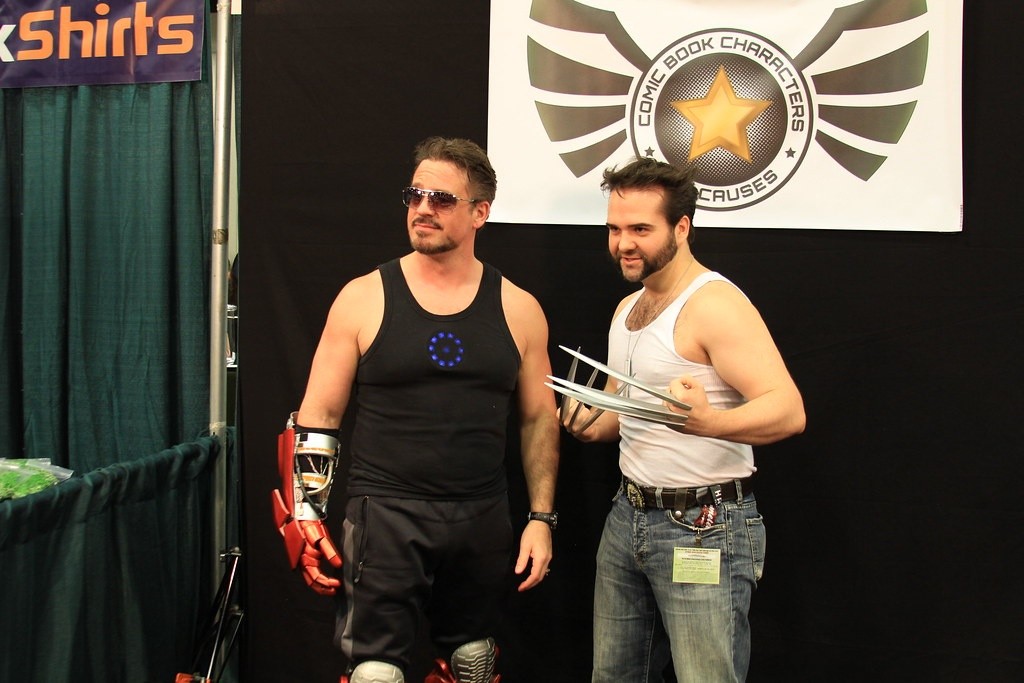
[624,256,693,398]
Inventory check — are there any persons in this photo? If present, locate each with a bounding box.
[274,137,559,683]
[557,155,806,682]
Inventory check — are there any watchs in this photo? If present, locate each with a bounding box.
[528,510,558,531]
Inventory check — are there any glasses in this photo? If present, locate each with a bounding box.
[402,186,477,212]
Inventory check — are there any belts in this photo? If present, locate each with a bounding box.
[623,475,754,509]
